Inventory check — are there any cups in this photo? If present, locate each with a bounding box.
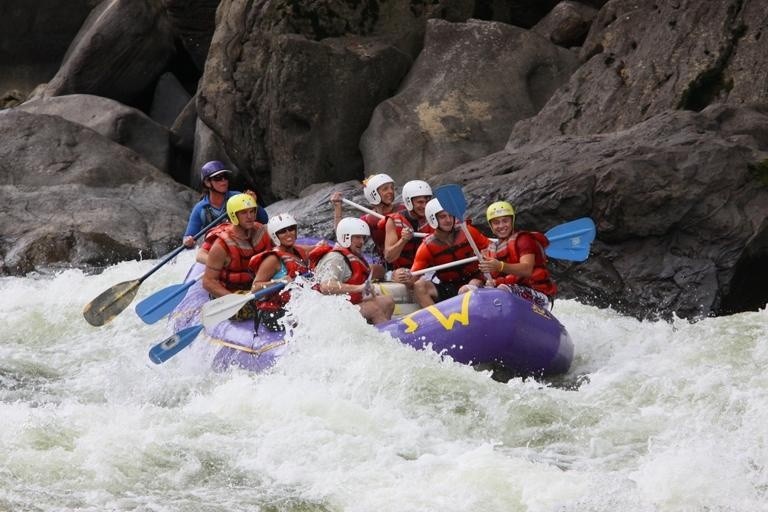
[278,226,296,234]
[210,175,231,181]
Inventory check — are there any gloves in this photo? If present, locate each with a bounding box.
[161,236,577,389]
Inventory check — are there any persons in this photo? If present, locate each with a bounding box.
[312,216,395,325]
[478,201,555,313]
[393,197,495,308]
[385,180,435,270]
[185,161,268,249]
[331,174,406,271]
[196,193,278,321]
[251,213,328,294]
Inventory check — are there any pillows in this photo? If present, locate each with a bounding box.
[402,178,434,212]
[200,159,233,186]
[336,215,371,249]
[267,212,298,247]
[486,200,516,235]
[226,192,258,226]
[363,173,396,206]
[424,197,445,230]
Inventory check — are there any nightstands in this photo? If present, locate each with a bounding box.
[149,318,204,364]
[410,218,596,276]
[136,271,206,324]
[84,211,228,326]
[200,272,312,327]
[413,232,590,261]
[434,185,492,284]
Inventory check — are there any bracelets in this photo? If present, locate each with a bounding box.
[499,261,504,272]
